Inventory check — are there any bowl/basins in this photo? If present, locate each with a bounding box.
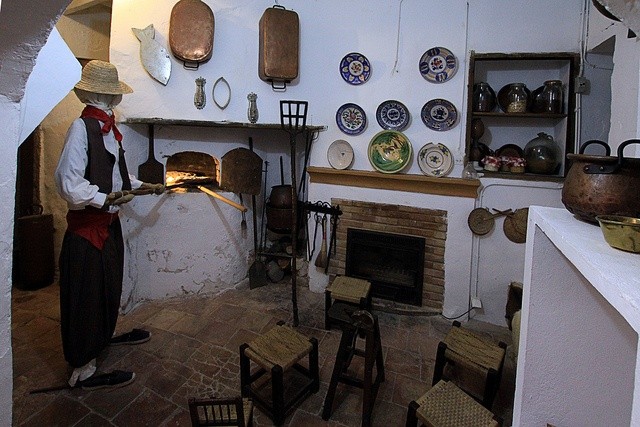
[595,214,640,253]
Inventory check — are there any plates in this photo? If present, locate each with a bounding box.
[336,102,366,135]
[421,98,458,131]
[417,141,453,177]
[375,99,410,130]
[327,139,354,169]
[419,46,458,83]
[367,129,412,173]
[339,52,370,85]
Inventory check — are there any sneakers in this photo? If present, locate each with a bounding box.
[82,370,136,391]
[109,328,152,346]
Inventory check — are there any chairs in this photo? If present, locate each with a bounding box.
[187,393,254,426]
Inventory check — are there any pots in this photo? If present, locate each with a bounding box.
[561,138,640,226]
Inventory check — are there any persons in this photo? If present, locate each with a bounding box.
[54,60,167,391]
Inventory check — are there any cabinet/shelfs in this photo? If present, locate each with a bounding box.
[119,116,325,133]
[463,47,580,182]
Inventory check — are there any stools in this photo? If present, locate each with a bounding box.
[406,376,505,427]
[324,273,373,331]
[432,320,508,411]
[238,319,320,426]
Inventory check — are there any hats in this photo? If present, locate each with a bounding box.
[75,60,133,95]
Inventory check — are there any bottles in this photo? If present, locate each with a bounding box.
[506,81,527,112]
[542,79,564,113]
[475,81,493,111]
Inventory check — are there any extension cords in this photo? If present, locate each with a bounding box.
[471,296,482,309]
